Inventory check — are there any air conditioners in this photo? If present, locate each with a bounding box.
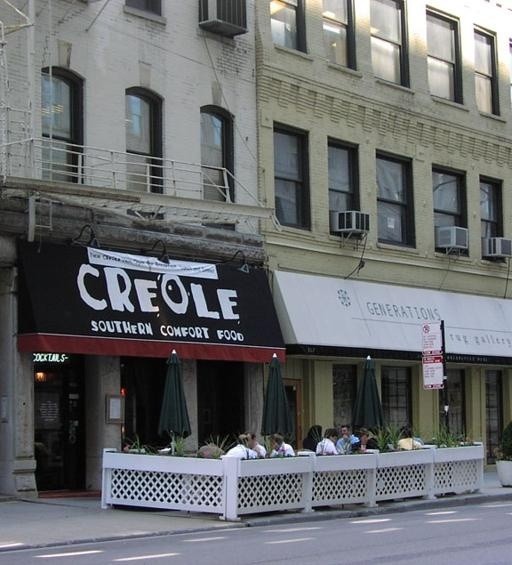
[331,210,371,235]
[435,226,468,249]
[482,236,512,258]
[199,0,249,38]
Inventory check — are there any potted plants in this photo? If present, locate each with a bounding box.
[496,420,512,486]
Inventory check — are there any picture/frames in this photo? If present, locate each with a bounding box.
[106,394,125,424]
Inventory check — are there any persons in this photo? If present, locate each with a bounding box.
[245,432,267,459]
[336,425,359,455]
[225,434,258,459]
[400,427,424,446]
[316,428,340,455]
[359,428,369,446]
[199,437,225,459]
[366,438,381,454]
[122,440,132,451]
[269,433,295,457]
[302,425,323,453]
